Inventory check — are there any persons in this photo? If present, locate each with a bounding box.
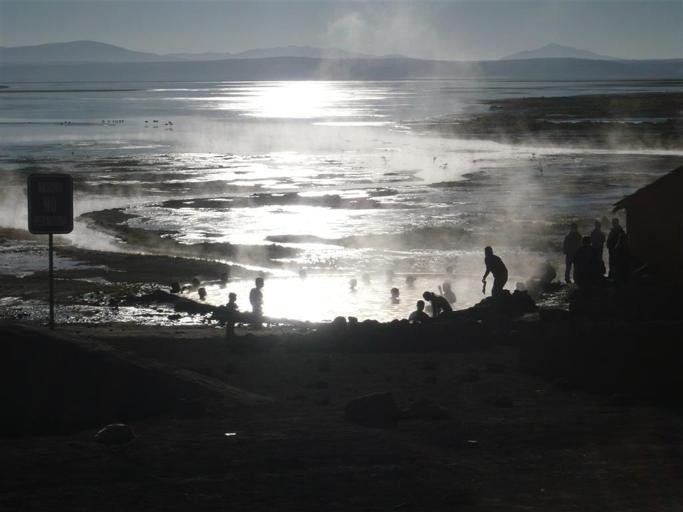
[348,269,395,289]
[404,277,417,291]
[191,278,200,287]
[390,288,400,298]
[444,266,456,281]
[226,292,238,308]
[409,282,457,322]
[563,218,627,284]
[197,288,207,299]
[249,278,264,315]
[482,246,509,297]
[221,272,228,282]
[169,281,180,293]
